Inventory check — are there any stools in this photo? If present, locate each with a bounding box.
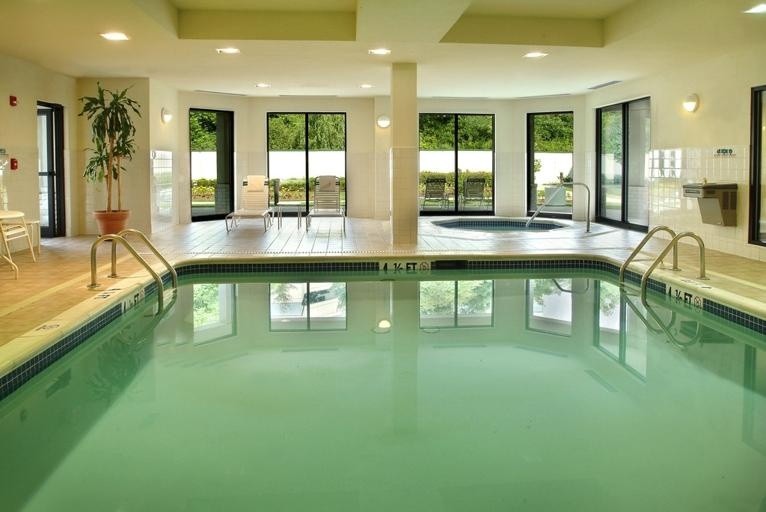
[8,220,40,257]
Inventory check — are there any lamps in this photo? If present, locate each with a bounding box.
[682,94,699,112]
[161,107,173,124]
[377,114,391,129]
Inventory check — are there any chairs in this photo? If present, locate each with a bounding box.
[225,174,274,234]
[305,175,345,235]
[0,210,36,268]
[458,177,486,209]
[421,178,449,209]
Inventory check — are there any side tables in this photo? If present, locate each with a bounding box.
[276,201,310,230]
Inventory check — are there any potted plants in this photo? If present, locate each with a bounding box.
[72,80,144,241]
